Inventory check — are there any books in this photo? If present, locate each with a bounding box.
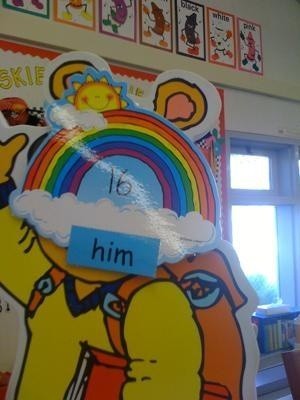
[257,303,296,353]
